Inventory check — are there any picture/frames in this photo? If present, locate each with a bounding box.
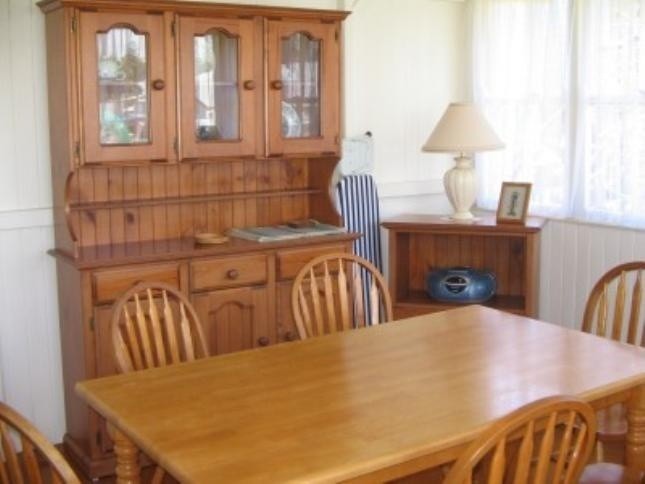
[495,181,532,225]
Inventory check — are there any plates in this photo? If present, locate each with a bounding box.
[282,101,302,138]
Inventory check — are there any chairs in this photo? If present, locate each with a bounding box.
[441,394,598,484]
[108,280,211,484]
[0,401,82,484]
[582,261,645,467]
[289,253,393,340]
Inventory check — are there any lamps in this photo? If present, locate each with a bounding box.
[422,102,504,223]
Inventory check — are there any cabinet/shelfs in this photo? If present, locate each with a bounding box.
[380,212,549,321]
